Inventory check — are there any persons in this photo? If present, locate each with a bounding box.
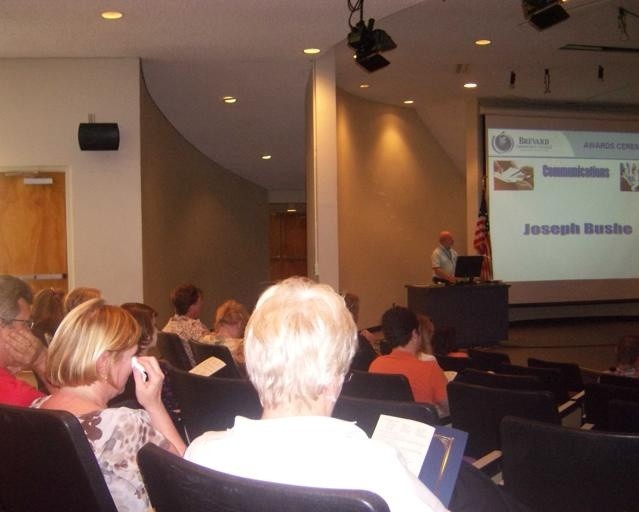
[430,230,471,287]
[602,335,639,378]
[1,276,454,511]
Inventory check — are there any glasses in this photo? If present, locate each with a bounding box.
[12,318,34,328]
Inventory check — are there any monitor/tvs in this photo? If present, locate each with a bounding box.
[454,256,484,284]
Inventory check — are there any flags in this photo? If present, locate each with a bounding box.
[470,181,493,285]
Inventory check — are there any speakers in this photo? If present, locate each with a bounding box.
[78,123,120,151]
